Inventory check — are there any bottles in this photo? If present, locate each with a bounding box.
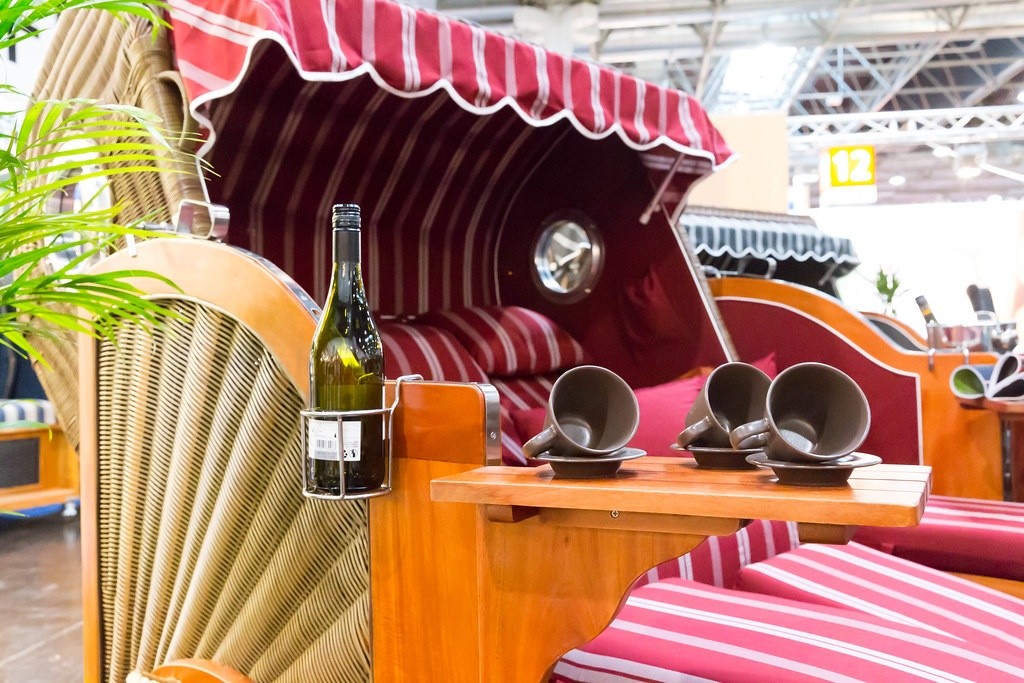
[916,286,1018,354]
[309,203,385,493]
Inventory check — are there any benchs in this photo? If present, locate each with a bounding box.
[71,243,1024,683]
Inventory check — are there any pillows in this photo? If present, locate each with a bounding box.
[511,352,778,460]
[380,322,489,380]
[408,303,591,377]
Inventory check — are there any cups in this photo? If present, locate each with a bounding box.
[730,362,871,462]
[677,362,774,448]
[522,365,639,459]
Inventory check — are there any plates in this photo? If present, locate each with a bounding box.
[527,448,646,477]
[745,452,882,486]
[671,443,766,470]
